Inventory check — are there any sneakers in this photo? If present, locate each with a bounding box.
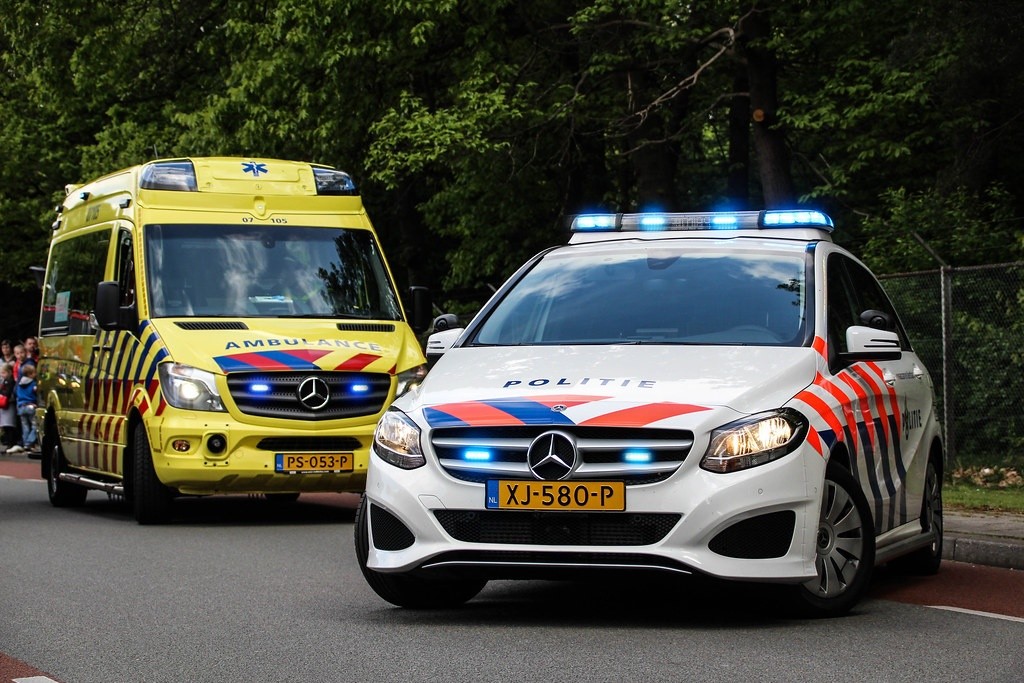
[6,445,25,454]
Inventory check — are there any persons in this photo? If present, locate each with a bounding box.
[0,364,23,453]
[0,340,17,364]
[17,364,39,452]
[13,345,26,382]
[24,338,39,362]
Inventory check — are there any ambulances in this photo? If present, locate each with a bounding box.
[37,155,459,525]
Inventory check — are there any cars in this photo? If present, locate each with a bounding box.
[355,211,944,615]
